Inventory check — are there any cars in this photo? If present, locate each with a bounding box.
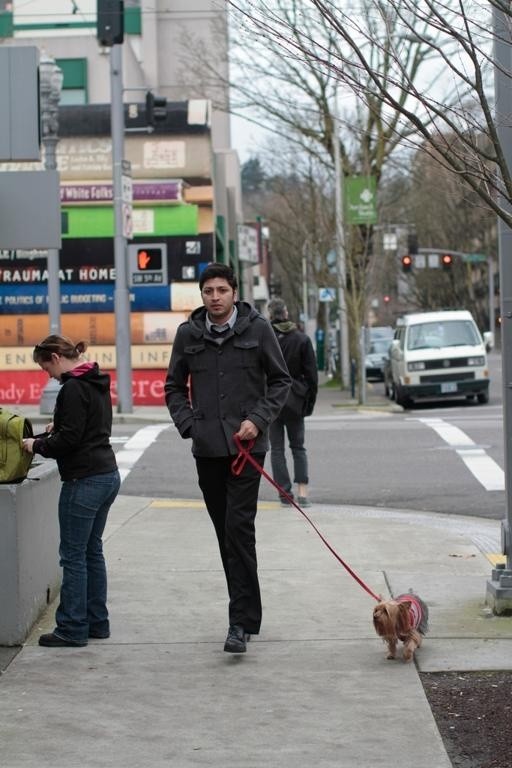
[329,325,394,379]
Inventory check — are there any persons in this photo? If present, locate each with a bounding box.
[265,298,315,507]
[164,263,293,654]
[20,335,124,646]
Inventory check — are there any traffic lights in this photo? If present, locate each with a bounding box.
[137,246,162,272]
[443,253,453,272]
[402,255,413,272]
[147,90,168,123]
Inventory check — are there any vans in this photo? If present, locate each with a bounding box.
[387,307,496,407]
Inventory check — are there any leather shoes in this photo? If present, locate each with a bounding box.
[38,632,110,647]
[224,624,251,652]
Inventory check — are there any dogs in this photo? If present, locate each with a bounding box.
[371,587,429,663]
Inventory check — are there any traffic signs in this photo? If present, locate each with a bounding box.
[460,252,487,262]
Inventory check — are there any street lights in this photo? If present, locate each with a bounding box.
[33,45,66,415]
[332,31,436,393]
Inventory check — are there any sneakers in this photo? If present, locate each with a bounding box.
[279,494,311,508]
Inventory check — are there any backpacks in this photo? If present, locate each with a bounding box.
[0,408,50,484]
